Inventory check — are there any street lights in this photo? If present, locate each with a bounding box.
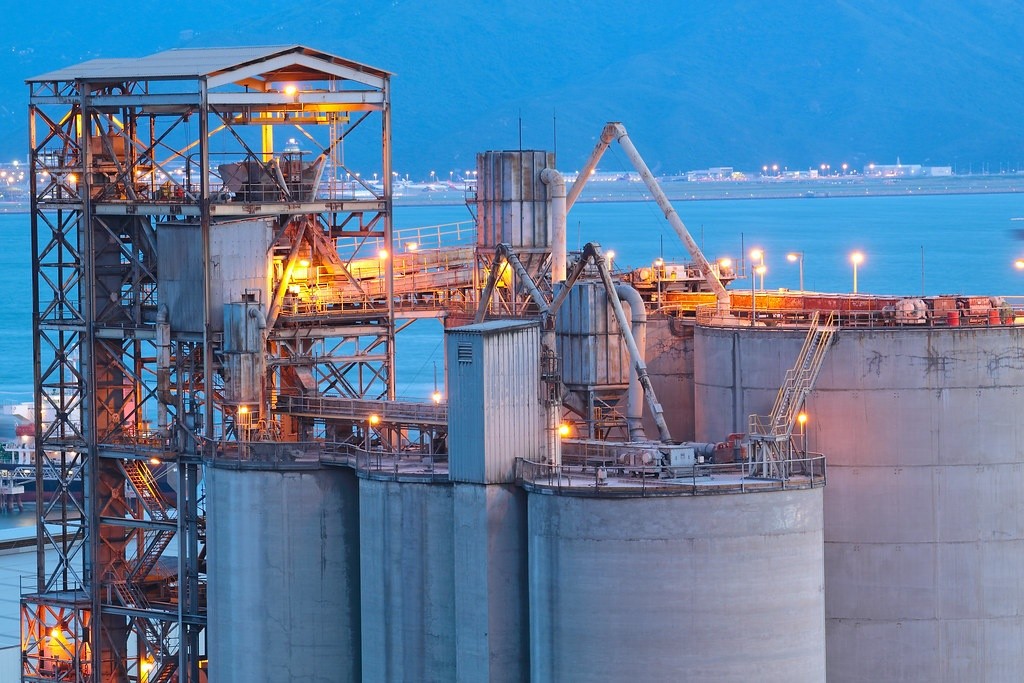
[750,248,764,289]
[367,411,379,463]
[798,413,807,474]
[751,264,766,326]
[655,257,664,307]
[850,253,865,293]
[787,250,805,291]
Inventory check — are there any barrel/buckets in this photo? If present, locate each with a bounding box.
[947,311,959,326]
[989,311,999,325]
